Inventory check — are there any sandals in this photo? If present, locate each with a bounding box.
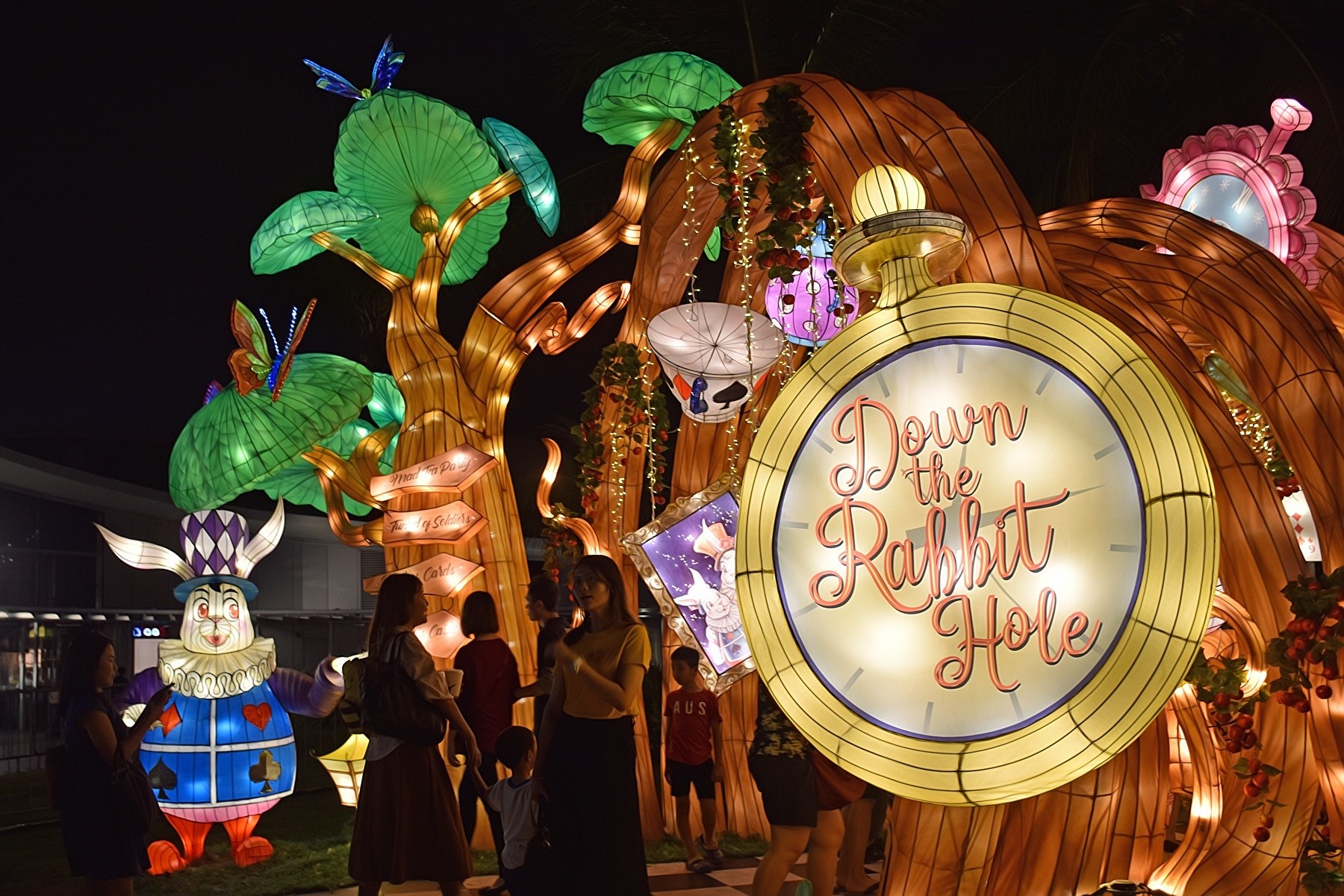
[686,855,712,874]
[702,842,725,862]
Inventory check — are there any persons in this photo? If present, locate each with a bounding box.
[747,674,879,896]
[664,646,726,871]
[347,554,652,896]
[48,632,175,896]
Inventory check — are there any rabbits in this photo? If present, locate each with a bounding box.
[95,495,296,874]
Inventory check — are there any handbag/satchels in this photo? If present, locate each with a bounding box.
[361,662,446,747]
[813,749,867,808]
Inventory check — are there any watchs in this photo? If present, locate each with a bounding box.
[568,656,584,676]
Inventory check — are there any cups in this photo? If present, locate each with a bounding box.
[444,669,464,697]
